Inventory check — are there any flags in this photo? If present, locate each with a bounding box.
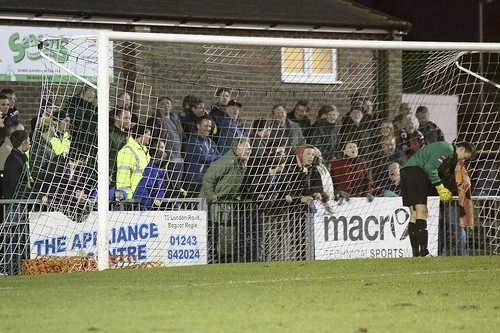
[455,160,474,230]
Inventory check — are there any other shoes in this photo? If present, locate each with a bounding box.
[412,253,434,257]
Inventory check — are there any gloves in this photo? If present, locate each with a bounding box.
[435,183,452,204]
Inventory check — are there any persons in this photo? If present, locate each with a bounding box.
[0,84,467,275]
[399,140,476,258]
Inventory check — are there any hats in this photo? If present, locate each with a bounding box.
[228,99,243,108]
